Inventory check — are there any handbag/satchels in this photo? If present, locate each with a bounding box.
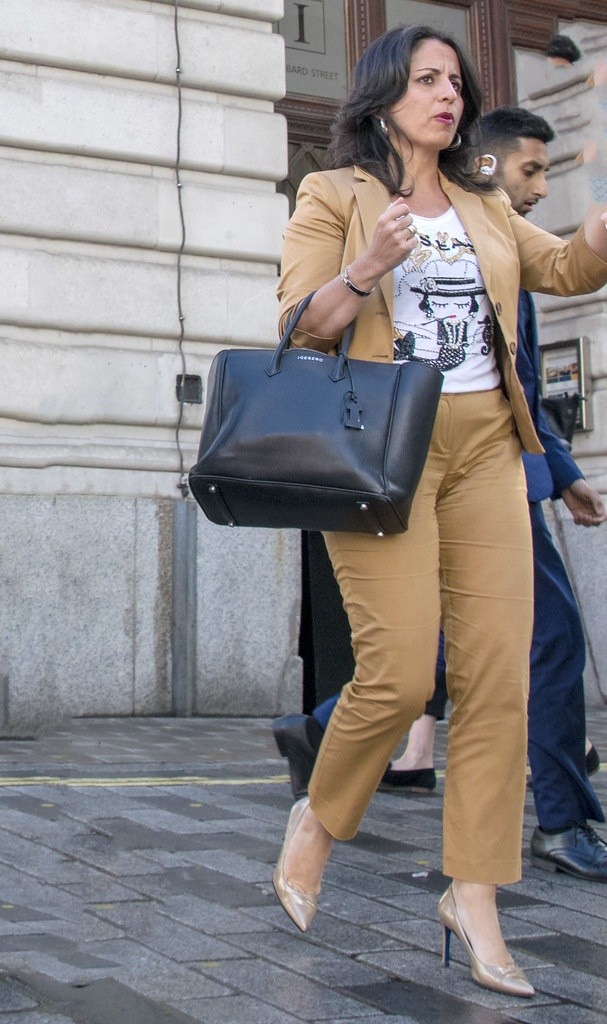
[188,288,444,538]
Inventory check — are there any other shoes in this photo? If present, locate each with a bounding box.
[526,745,600,790]
[376,761,436,794]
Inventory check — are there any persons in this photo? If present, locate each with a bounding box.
[274,21,607,995]
[274,105,607,884]
[370,625,600,795]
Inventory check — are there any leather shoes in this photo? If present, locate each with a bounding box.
[530,820,607,884]
[272,713,317,803]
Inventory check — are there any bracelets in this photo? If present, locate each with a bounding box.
[342,266,377,297]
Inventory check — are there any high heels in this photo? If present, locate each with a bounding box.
[272,797,335,933]
[437,883,536,998]
